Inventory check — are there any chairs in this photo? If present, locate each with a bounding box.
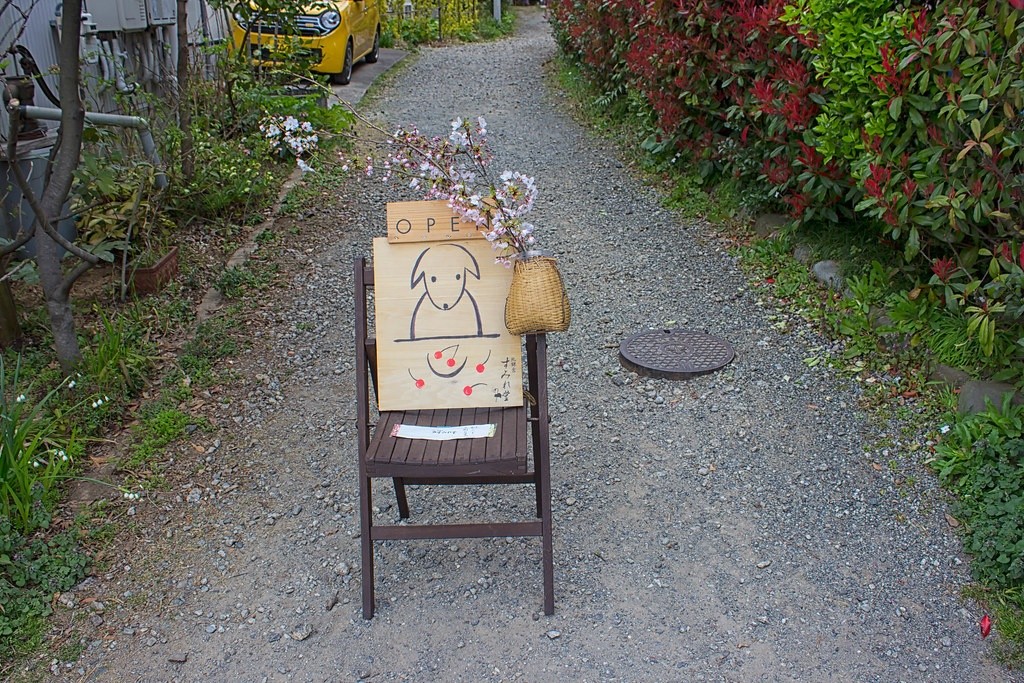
[353,200,570,620]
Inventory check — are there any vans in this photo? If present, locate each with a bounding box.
[227,0,381,85]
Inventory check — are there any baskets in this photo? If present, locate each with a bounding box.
[503,256,571,337]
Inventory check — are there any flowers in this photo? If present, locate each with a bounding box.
[259,74,539,268]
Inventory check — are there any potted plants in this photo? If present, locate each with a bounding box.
[124,245,179,294]
